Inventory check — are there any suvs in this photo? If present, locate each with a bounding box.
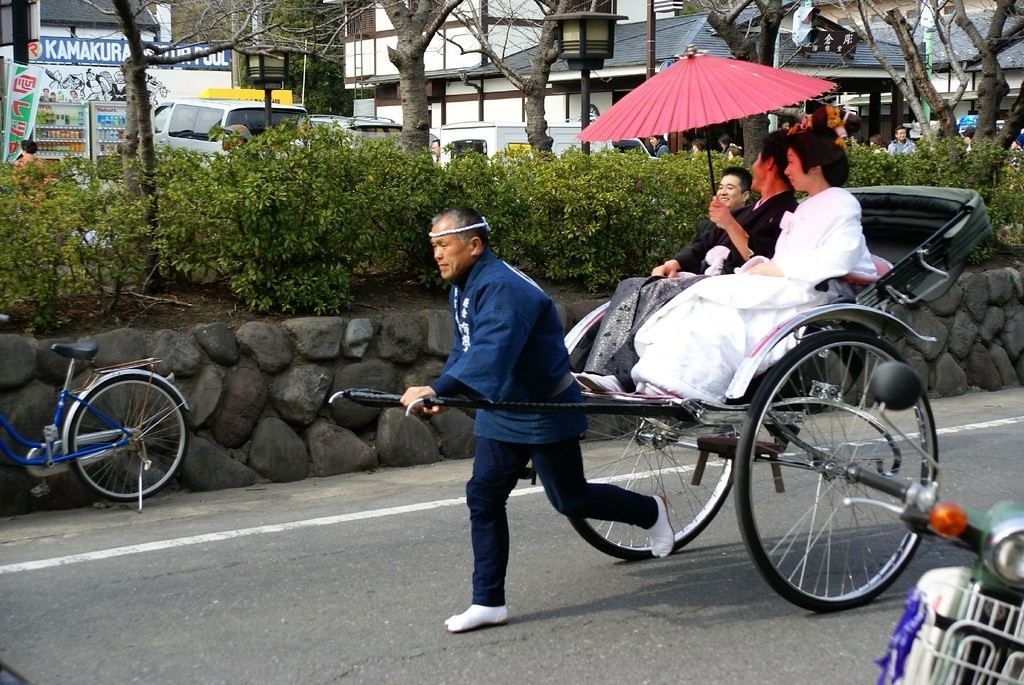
[151,97,402,178]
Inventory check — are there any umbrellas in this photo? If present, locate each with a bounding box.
[577,51,838,142]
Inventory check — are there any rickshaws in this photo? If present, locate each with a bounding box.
[328,185,996,613]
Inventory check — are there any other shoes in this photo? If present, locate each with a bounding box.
[646,495,675,558]
[444,604,509,633]
[570,371,593,391]
[576,374,623,393]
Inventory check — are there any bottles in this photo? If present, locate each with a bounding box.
[36,110,84,152]
[101,116,125,139]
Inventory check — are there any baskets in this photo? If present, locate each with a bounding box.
[911,582,1024,685]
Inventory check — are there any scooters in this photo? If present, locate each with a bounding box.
[841,360,1024,685]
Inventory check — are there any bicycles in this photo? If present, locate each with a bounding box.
[0,314,192,514]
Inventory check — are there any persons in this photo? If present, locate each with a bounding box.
[963,127,1024,153]
[870,134,887,153]
[222,125,252,153]
[70,91,78,100]
[649,135,670,158]
[400,205,675,633]
[14,140,47,184]
[692,139,706,161]
[887,126,915,155]
[632,106,875,406]
[39,89,50,102]
[697,167,752,237]
[432,139,440,162]
[570,129,799,393]
[50,92,57,102]
[718,134,742,160]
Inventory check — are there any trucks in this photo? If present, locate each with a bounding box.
[428,120,661,180]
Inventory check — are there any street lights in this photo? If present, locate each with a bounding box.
[544,10,629,204]
[245,44,289,129]
